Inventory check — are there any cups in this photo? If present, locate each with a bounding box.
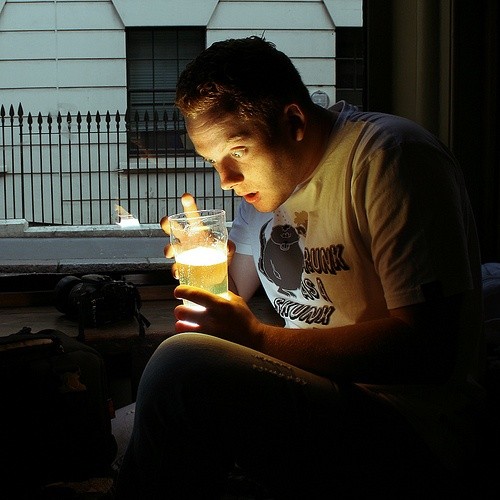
[166,208,230,310]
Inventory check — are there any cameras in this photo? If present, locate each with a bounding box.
[58,275,141,325]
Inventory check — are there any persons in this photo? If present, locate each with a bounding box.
[92,32,482,500]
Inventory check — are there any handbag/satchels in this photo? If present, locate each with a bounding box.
[1,328,118,489]
